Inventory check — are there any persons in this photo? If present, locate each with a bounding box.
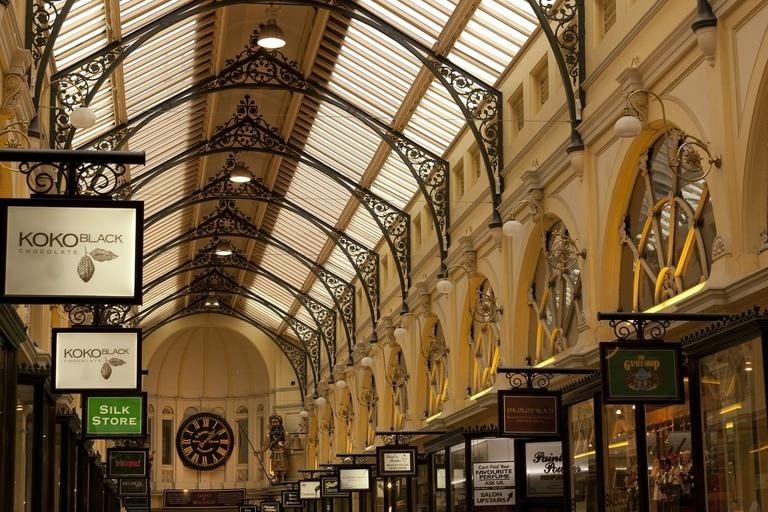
[254,413,290,484]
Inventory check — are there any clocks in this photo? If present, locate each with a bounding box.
[177,413,236,471]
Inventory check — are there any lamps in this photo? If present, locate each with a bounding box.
[299,343,410,447]
[230,165,252,184]
[436,264,503,323]
[393,313,449,362]
[256,18,286,50]
[216,242,233,256]
[205,297,220,307]
[0,78,96,172]
[614,88,722,182]
[503,199,586,270]
